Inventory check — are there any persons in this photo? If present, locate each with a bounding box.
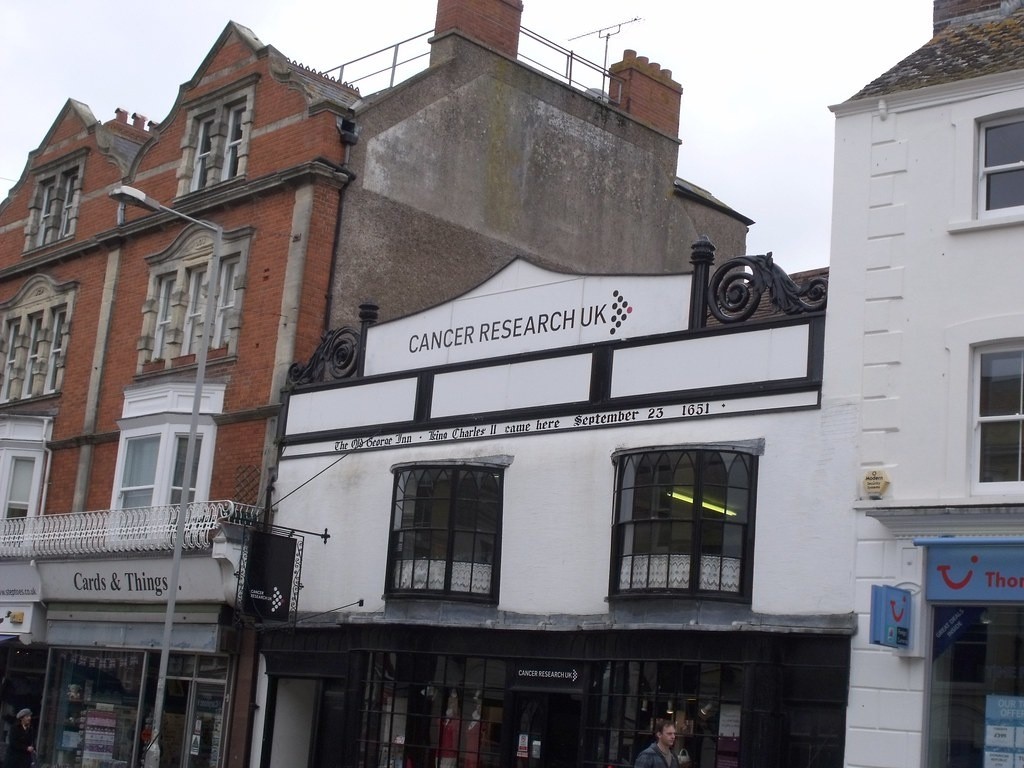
[634,720,680,768]
[435,708,460,768]
[3,708,35,768]
[465,710,487,768]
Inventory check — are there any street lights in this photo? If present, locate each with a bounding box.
[104,182,223,768]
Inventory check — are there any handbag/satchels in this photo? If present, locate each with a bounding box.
[677,749,690,764]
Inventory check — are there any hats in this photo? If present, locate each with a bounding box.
[16,708,33,719]
